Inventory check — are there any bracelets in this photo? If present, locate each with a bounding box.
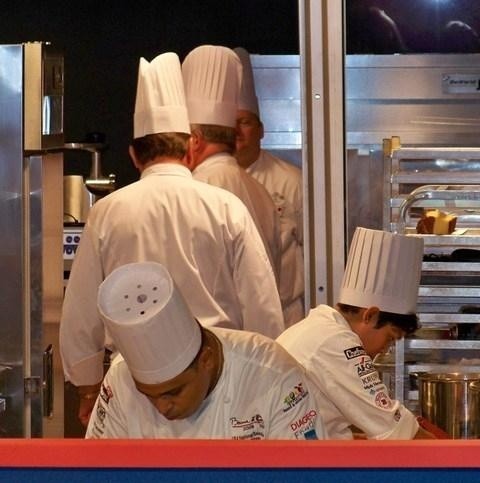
[79,391,98,399]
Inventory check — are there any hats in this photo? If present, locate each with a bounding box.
[133,51,192,141]
[338,228,423,315]
[233,48,260,118]
[96,260,202,386]
[180,44,244,129]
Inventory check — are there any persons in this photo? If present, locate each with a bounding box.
[86,318,330,441]
[182,124,285,301]
[56,132,286,430]
[273,302,453,440]
[229,109,308,325]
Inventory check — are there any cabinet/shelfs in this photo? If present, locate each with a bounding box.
[395,184,479,405]
[382,135,479,232]
